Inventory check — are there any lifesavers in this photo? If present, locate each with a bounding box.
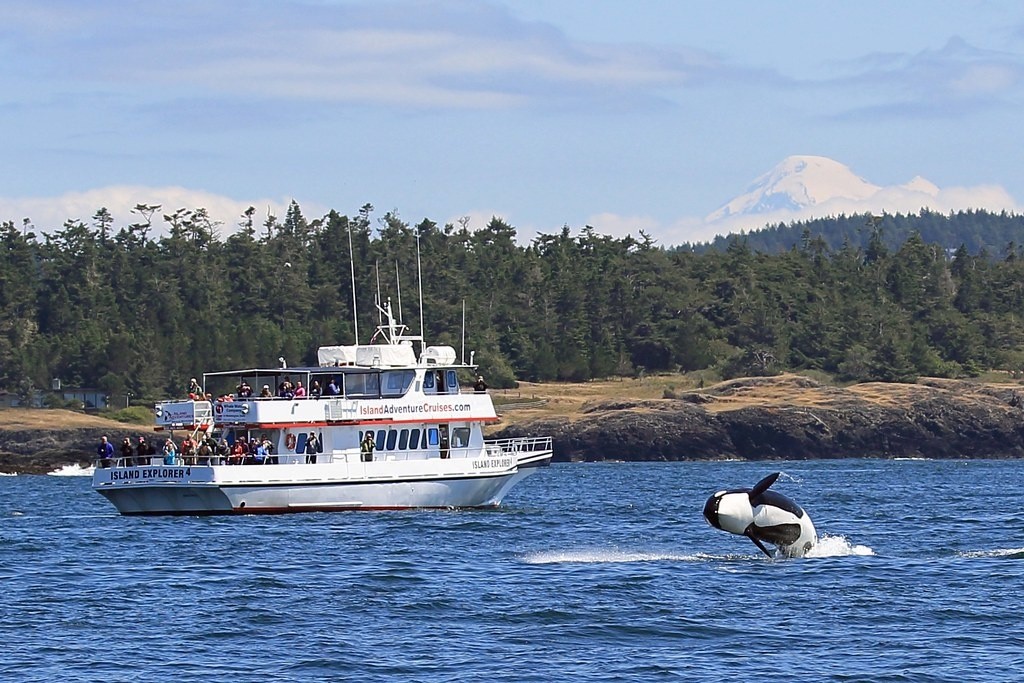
[285,432,296,449]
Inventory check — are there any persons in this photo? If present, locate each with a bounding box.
[310,379,323,399]
[360,434,377,462]
[186,378,214,415]
[180,432,275,466]
[136,436,151,465]
[162,436,179,465]
[260,385,272,401]
[216,380,253,426]
[305,431,321,464]
[473,375,489,394]
[279,381,305,400]
[120,436,134,467]
[328,375,340,395]
[452,431,463,448]
[96,435,115,468]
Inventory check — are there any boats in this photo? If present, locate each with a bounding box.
[89,219,554,514]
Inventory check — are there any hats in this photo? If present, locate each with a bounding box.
[263,385,269,388]
[191,379,196,383]
[251,438,255,440]
